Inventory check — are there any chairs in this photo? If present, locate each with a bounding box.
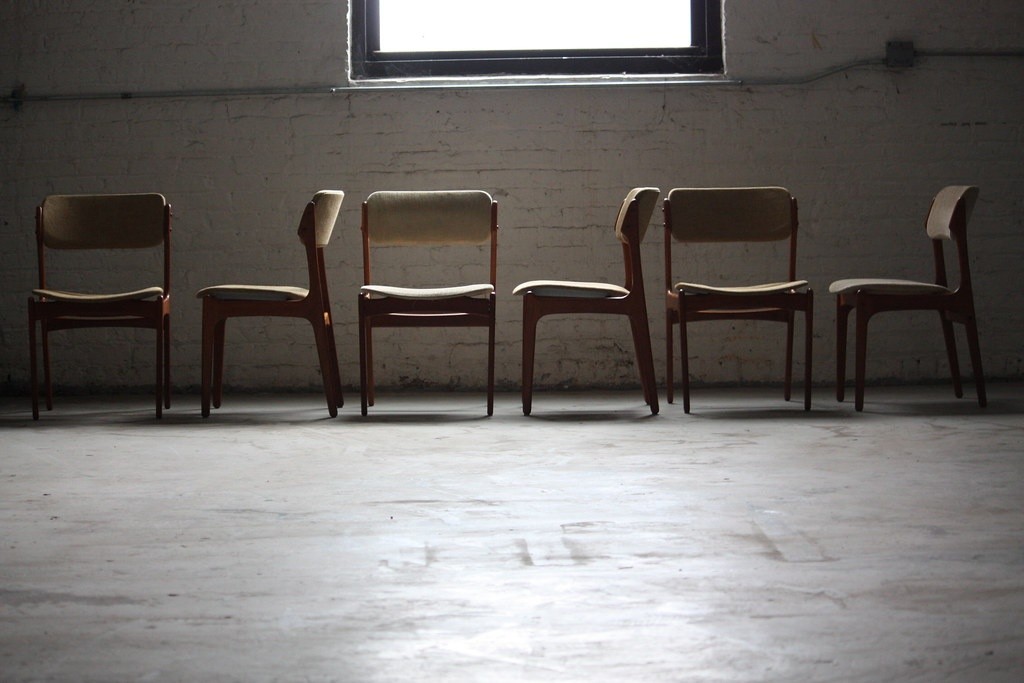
[828,185,987,411]
[359,190,499,414]
[664,187,813,414]
[195,189,344,418]
[27,192,171,417]
[514,187,659,415]
